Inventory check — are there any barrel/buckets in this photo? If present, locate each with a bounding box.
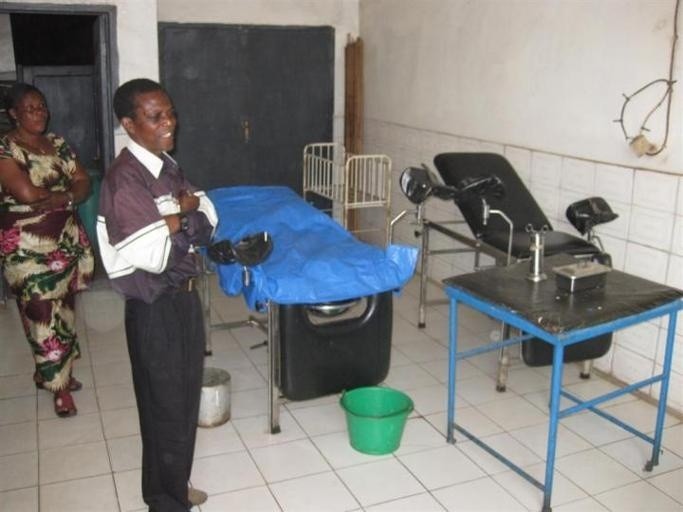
[340,385,415,457]
[196,366,232,429]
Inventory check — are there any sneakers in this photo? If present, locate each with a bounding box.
[187,488,207,506]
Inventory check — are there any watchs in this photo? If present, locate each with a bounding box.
[176,213,189,234]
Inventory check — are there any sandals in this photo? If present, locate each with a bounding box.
[53,388,77,418]
[33,372,82,392]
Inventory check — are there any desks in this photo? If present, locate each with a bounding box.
[438,246,683,512]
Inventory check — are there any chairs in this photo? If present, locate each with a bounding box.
[388,150,617,396]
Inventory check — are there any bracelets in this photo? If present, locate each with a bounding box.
[64,190,74,207]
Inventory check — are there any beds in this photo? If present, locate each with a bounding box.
[192,183,404,438]
[303,141,396,248]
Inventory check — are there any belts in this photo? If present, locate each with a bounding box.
[170,278,197,293]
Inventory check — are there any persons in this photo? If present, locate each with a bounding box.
[92,75,220,512]
[0,82,95,421]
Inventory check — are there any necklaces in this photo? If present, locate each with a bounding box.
[14,130,46,156]
